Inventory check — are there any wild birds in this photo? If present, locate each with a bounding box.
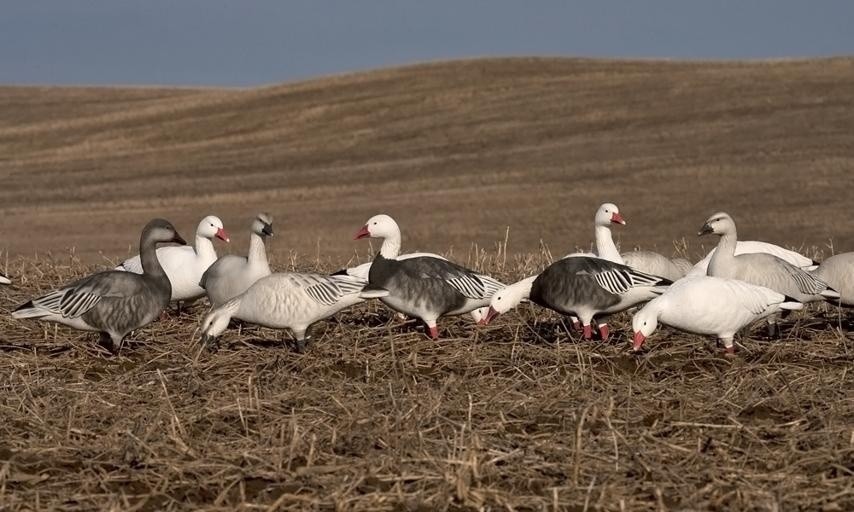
[485,203,854,353]
[199,210,276,311]
[332,213,507,342]
[111,215,232,315]
[198,270,389,353]
[9,218,188,358]
